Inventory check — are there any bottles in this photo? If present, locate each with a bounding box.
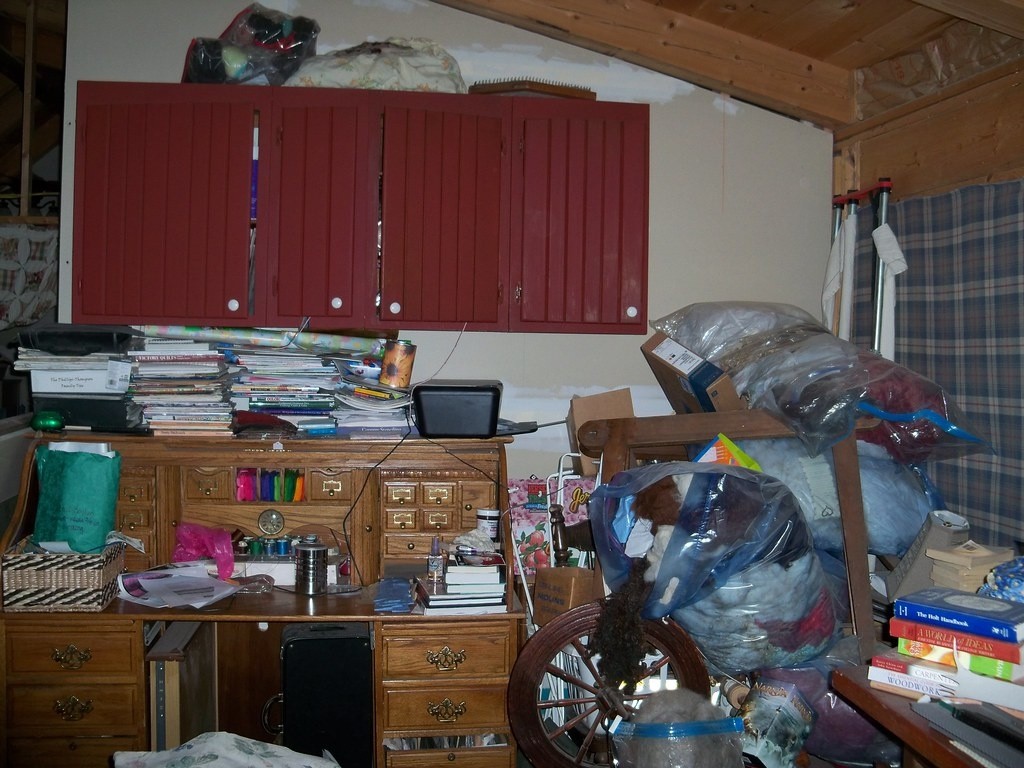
[428,536,443,580]
[476,509,500,543]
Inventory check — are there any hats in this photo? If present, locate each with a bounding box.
[228,410,299,434]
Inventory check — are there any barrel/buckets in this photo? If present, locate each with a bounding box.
[295,542,328,596]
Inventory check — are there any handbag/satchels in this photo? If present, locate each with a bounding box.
[507,478,596,575]
[35,445,122,554]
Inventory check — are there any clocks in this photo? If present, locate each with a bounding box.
[258,510,285,535]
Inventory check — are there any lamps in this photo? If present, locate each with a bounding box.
[883,509,970,645]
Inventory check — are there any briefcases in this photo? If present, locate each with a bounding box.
[262,623,374,768]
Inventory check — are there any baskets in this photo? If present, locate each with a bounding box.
[1,533,125,612]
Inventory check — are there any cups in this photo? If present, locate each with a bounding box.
[277,540,289,555]
[250,540,261,554]
[264,540,276,556]
[379,341,417,388]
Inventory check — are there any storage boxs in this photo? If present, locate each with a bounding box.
[2,534,127,612]
[262,623,374,768]
[640,332,742,417]
[733,676,816,768]
[565,386,635,476]
[532,566,594,628]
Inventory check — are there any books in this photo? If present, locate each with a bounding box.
[13,336,419,441]
[414,552,509,616]
[867,539,1024,712]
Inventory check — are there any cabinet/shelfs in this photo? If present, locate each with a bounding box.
[71,81,650,335]
[0,433,526,768]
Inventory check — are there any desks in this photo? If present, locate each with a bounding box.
[829,664,1024,768]
